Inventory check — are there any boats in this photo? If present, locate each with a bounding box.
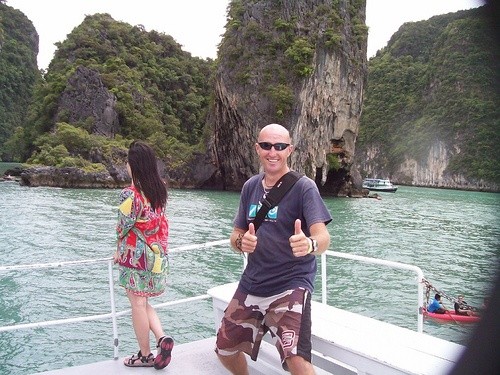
[424,304,482,324]
[362,178,399,192]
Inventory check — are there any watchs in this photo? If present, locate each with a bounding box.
[309,237,318,253]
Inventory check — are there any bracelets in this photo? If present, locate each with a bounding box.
[234,233,247,259]
[306,237,312,254]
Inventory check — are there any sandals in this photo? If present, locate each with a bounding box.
[124,350,156,367]
[154,335,174,370]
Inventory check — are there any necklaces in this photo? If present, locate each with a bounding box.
[263,177,274,193]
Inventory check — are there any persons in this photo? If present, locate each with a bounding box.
[428,294,451,314]
[454,295,479,316]
[113,140,174,370]
[214,124,333,375]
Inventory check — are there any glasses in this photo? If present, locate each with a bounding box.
[257,142,291,151]
